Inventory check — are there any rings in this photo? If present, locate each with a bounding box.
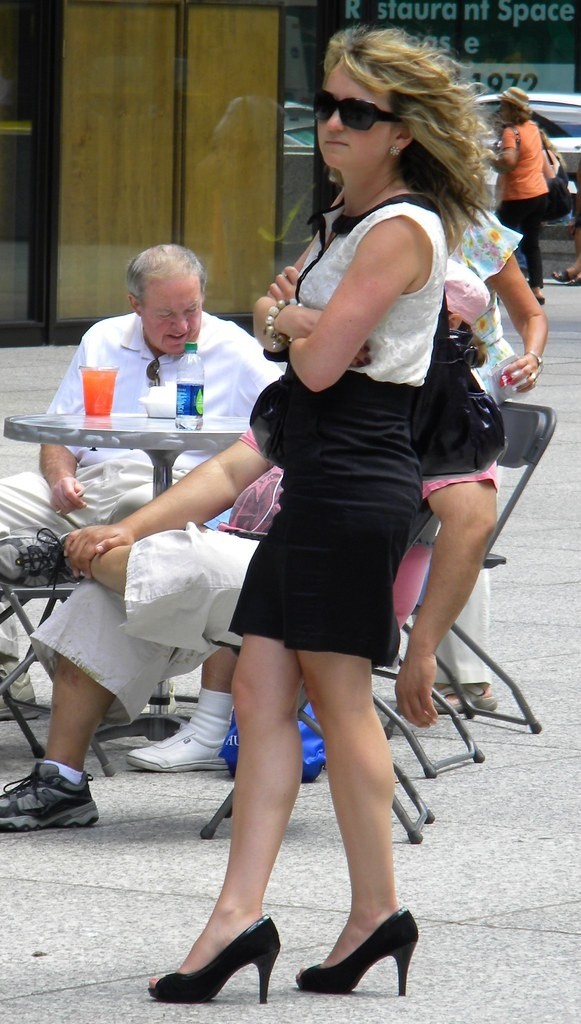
[531,373,537,380]
[56,509,61,513]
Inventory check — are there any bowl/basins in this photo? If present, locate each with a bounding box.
[139,385,177,419]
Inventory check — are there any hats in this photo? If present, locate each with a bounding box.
[498,87,531,112]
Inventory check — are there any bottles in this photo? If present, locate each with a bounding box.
[176,342,205,431]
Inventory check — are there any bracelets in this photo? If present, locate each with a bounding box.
[265,296,303,347]
[523,350,544,370]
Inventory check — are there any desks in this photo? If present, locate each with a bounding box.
[4,412,249,743]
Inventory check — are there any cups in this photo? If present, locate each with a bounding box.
[79,365,118,424]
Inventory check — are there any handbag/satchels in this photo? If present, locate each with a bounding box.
[408,328,509,478]
[540,178,571,221]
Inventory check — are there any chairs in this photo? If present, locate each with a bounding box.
[0,582,114,777]
[200,400,557,844]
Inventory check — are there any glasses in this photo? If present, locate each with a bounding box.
[147,357,160,387]
[312,89,402,130]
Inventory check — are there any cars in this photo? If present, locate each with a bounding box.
[283,92,581,221]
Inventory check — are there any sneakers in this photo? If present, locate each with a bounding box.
[0,668,40,719]
[0,762,99,829]
[0,528,84,600]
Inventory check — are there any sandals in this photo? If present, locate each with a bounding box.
[419,680,498,713]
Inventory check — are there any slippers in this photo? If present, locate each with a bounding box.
[567,275,581,286]
[536,298,546,305]
[551,270,573,281]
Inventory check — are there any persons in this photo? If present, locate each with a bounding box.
[480,85,557,306]
[0,260,499,830]
[212,76,290,185]
[552,153,581,286]
[125,636,241,771]
[148,22,549,1005]
[0,244,285,717]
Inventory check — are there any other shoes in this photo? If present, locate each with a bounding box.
[125,724,228,773]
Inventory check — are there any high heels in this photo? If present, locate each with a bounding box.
[147,913,280,1005]
[295,905,418,996]
